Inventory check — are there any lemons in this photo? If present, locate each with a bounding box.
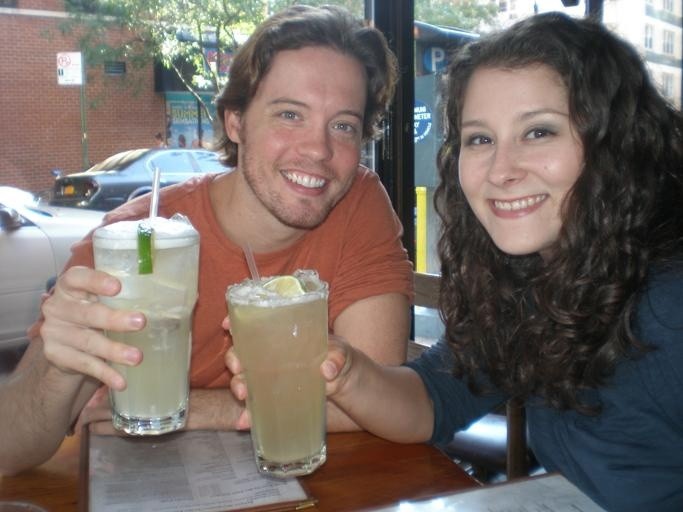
[262,273,307,300]
[136,222,155,276]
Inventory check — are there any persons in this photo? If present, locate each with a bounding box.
[178,133,187,149]
[155,132,167,148]
[220,13,683,512]
[191,138,200,149]
[0,5,414,478]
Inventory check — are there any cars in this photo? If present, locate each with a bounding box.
[0,185,112,358]
[48,149,240,213]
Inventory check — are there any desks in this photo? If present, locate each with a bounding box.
[3,416,486,512]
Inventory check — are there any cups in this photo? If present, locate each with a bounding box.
[224,278,328,478]
[93,227,201,437]
